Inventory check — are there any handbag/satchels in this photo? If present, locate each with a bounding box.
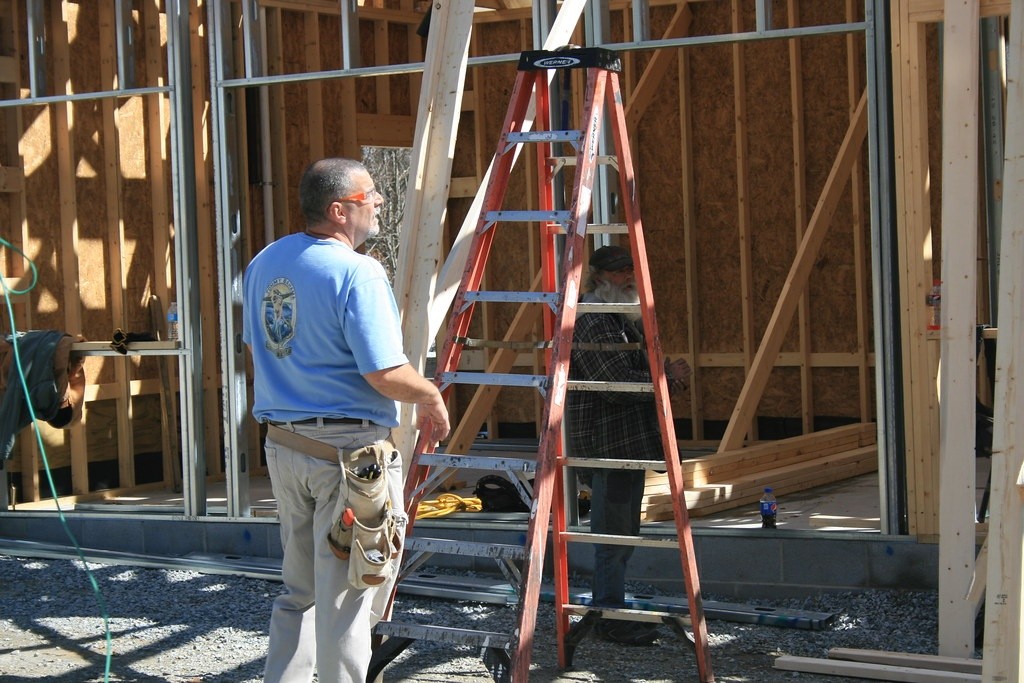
[328,444,401,589]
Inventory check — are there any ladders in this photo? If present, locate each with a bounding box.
[366,48,714,682]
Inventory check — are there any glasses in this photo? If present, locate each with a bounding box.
[326,187,376,215]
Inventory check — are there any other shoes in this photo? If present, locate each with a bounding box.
[595,620,662,647]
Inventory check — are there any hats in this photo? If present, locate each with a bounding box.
[589,246,633,271]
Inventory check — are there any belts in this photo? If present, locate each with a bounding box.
[266,415,379,425]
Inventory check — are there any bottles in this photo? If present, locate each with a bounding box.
[759,488,778,530]
[167,303,178,342]
[512,534,528,582]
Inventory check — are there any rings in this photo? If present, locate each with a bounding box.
[435,428,439,431]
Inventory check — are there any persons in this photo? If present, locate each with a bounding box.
[564,245,694,641]
[242,159,451,683]
